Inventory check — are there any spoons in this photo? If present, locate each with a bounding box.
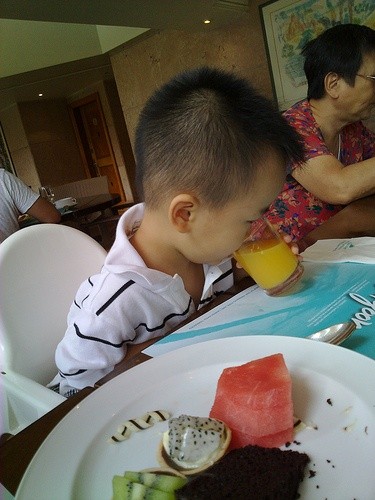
[304,322,357,345]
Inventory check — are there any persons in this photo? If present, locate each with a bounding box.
[246,23,375,243]
[0,168,61,244]
[44,68,307,397]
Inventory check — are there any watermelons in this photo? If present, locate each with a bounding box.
[208,353,295,452]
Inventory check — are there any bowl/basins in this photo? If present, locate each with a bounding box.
[52,196,76,210]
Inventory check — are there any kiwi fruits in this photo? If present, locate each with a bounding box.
[112,471,188,500]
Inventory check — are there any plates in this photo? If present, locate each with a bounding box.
[58,203,77,216]
[16,334,373,500]
[18,213,30,222]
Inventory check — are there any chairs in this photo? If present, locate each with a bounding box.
[98,201,134,246]
[0,224,107,433]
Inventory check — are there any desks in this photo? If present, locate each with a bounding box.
[0,193,375,495]
[22,194,120,231]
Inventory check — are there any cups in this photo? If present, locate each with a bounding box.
[230,217,303,298]
[37,184,56,205]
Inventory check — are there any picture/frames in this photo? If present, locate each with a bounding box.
[259,0,375,112]
[0,122,16,176]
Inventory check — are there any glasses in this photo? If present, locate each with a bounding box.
[355,72,375,81]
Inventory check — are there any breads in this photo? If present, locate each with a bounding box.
[174,444,311,500]
